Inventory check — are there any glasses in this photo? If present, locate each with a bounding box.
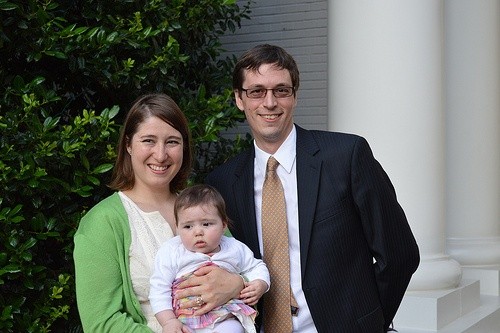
[240,86,295,98]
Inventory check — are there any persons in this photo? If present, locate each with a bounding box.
[147,183,271,333]
[201,43,420,333]
[72,92,258,333]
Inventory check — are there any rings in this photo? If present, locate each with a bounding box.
[195,296,205,307]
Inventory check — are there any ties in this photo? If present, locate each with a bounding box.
[262,155,299,333]
